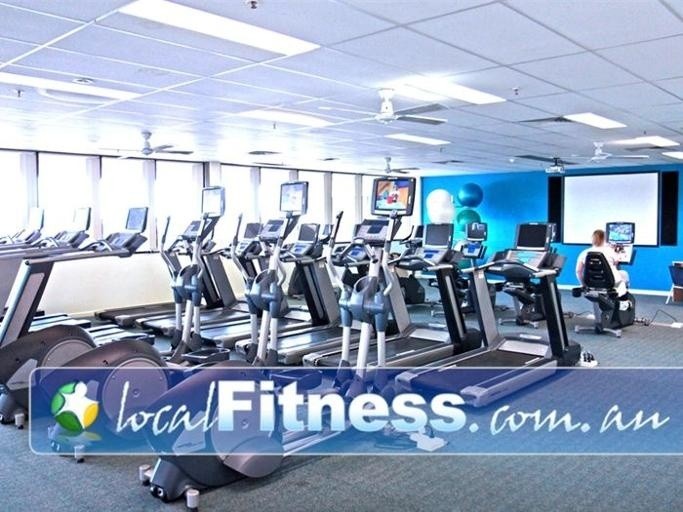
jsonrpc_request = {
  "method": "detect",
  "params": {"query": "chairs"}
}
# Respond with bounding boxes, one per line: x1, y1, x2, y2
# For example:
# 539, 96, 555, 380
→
665, 266, 683, 305
572, 252, 622, 338
498, 259, 539, 329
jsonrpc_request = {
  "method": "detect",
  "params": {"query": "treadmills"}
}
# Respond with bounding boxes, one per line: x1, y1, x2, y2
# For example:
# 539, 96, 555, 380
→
300, 222, 483, 390
394, 221, 581, 413
135, 183, 375, 369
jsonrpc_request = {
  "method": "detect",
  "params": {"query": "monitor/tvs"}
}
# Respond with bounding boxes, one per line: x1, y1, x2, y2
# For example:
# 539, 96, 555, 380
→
28, 207, 44, 229
179, 176, 636, 266
72, 207, 91, 230
125, 207, 148, 233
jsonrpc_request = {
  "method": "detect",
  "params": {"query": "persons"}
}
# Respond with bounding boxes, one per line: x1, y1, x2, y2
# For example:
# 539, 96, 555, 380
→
387, 184, 399, 204
576, 230, 630, 298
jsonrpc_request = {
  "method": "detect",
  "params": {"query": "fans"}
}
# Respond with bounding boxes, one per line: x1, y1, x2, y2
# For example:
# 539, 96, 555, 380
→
309, 88, 450, 134
559, 141, 649, 165
366, 156, 420, 175
99, 132, 193, 161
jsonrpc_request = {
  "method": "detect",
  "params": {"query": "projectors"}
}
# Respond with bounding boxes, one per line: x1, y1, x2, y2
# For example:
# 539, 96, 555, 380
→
545, 164, 565, 174
